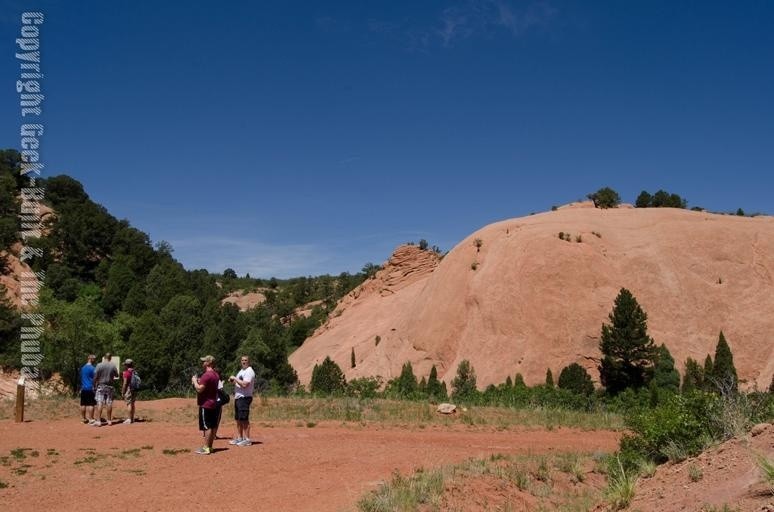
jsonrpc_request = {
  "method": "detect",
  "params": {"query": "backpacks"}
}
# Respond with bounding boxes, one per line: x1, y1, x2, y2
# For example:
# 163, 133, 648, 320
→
130, 370, 141, 390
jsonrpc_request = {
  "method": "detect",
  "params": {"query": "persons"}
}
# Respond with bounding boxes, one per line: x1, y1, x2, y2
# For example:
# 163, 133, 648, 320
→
228, 355, 256, 446
190, 354, 223, 456
92, 352, 118, 428
201, 369, 225, 440
79, 354, 96, 426
120, 358, 138, 424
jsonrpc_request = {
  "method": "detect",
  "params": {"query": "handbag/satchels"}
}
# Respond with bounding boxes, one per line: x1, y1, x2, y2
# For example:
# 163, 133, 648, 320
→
218, 387, 230, 407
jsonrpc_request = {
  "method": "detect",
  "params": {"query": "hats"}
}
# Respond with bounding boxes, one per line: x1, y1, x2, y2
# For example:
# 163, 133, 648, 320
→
122, 358, 135, 365
200, 355, 215, 362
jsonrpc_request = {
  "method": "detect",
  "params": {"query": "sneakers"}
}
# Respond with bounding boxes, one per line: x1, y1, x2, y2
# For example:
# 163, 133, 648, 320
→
229, 438, 252, 447
81, 417, 135, 427
194, 445, 213, 455
